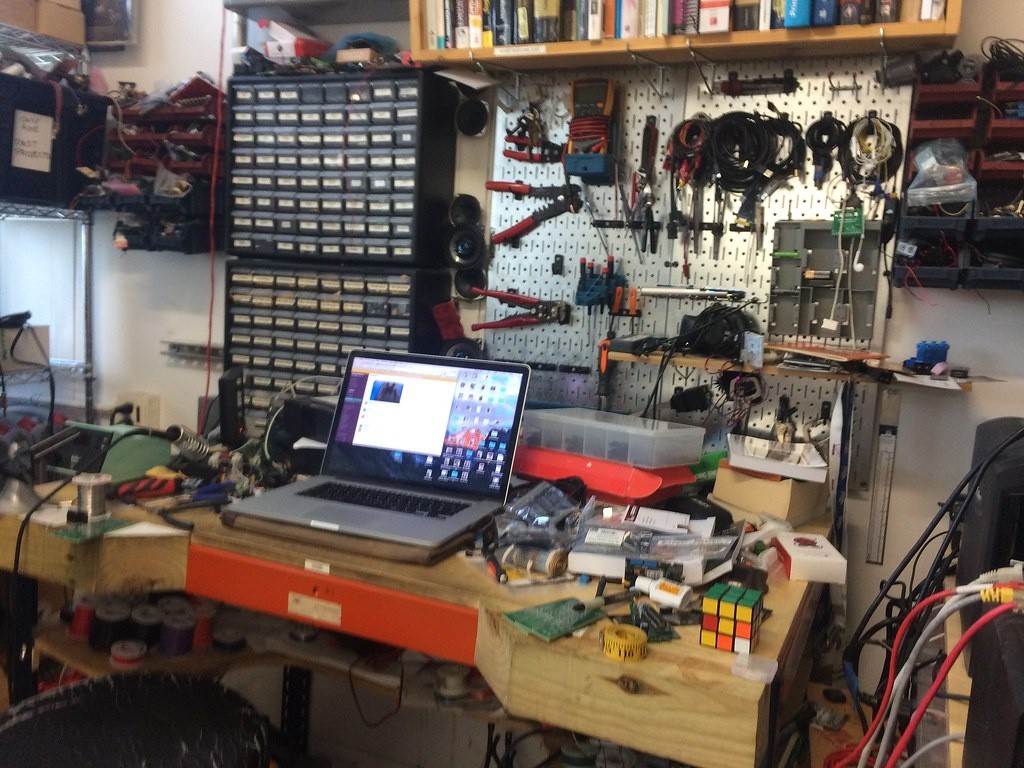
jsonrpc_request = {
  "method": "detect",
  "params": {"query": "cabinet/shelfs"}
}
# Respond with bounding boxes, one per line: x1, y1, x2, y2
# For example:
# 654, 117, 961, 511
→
0, 484, 835, 768
889, 67, 1024, 290
409, 0, 960, 73
0, 72, 93, 426
223, 67, 457, 470
104, 78, 223, 255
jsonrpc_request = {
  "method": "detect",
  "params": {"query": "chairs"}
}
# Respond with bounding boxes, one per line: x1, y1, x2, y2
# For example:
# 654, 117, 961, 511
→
0, 668, 275, 768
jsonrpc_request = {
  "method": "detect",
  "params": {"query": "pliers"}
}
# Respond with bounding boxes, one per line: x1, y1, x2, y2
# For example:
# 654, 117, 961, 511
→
502, 135, 562, 163
468, 287, 571, 332
485, 180, 584, 244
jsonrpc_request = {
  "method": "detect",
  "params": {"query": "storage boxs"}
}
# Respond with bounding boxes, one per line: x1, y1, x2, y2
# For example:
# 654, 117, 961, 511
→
712, 460, 829, 521
770, 531, 847, 587
0, 325, 49, 373
0, 0, 87, 45
512, 443, 696, 508
523, 406, 706, 470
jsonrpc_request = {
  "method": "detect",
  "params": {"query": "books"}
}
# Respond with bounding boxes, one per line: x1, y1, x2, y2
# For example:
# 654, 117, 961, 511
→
426, 0, 947, 50
568, 504, 746, 587
726, 433, 828, 483
764, 342, 890, 362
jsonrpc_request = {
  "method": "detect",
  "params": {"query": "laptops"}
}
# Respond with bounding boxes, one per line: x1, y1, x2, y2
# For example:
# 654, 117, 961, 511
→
223, 346, 531, 550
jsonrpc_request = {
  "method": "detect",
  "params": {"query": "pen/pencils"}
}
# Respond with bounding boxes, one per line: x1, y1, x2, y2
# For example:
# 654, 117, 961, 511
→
481, 546, 509, 584
570, 590, 641, 612
594, 576, 607, 597
